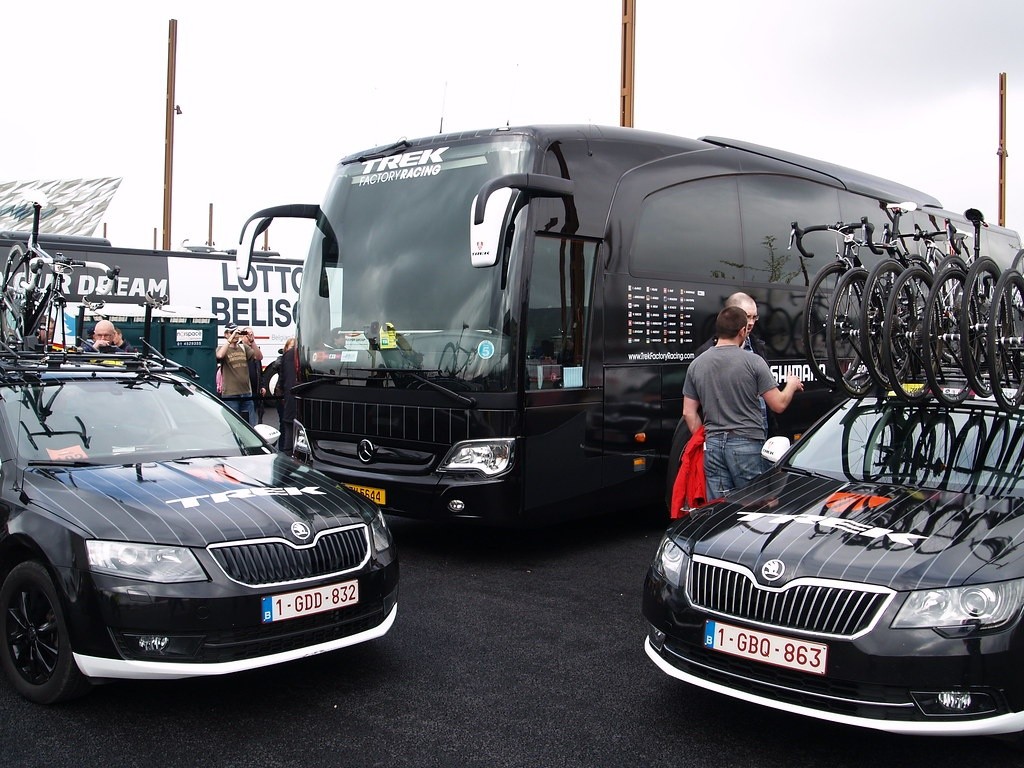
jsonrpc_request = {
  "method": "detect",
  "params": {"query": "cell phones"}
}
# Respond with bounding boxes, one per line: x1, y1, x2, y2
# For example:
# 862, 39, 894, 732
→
239, 331, 248, 335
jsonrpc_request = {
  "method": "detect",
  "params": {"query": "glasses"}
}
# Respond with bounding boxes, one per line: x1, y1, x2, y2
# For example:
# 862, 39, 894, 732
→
747, 315, 759, 321
229, 332, 233, 335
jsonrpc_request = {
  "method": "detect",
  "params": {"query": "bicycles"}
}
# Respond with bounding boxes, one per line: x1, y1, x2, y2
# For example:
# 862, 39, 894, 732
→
808, 395, 1024, 554
7, 383, 92, 451
0, 232, 121, 346
788, 201, 1024, 414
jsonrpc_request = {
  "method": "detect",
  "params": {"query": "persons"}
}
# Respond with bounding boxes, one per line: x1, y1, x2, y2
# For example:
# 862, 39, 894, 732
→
682, 306, 804, 503
37, 316, 55, 344
281, 348, 296, 457
80, 320, 124, 355
329, 328, 347, 349
694, 292, 778, 440
112, 327, 137, 353
215, 323, 263, 428
260, 338, 295, 452
238, 327, 265, 424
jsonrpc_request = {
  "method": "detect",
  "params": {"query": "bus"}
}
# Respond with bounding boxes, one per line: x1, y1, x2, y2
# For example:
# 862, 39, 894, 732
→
236, 123, 1023, 563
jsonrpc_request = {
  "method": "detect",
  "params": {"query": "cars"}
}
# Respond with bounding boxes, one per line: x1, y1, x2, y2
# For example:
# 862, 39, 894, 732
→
0, 352, 398, 705
640, 386, 1024, 739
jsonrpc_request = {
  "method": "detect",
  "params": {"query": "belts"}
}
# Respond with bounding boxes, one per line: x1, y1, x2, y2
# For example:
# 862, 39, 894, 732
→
709, 432, 744, 439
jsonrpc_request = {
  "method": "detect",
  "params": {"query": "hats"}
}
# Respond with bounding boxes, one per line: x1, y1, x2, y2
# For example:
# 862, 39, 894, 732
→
86, 325, 95, 335
224, 323, 237, 333
